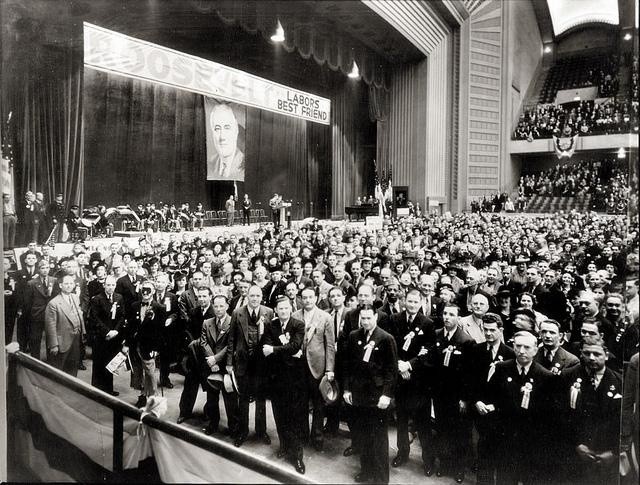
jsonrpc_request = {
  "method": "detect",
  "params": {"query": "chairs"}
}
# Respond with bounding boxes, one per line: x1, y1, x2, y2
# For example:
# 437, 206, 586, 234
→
222, 211, 228, 225
217, 211, 228, 226
234, 210, 241, 226
250, 209, 257, 223
211, 211, 221, 226
203, 216, 209, 226
240, 210, 248, 225
205, 211, 215, 226
260, 209, 268, 222
255, 209, 263, 222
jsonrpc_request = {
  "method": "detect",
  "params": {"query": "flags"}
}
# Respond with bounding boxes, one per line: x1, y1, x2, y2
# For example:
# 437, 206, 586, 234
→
0, 121, 14, 200
373, 165, 392, 212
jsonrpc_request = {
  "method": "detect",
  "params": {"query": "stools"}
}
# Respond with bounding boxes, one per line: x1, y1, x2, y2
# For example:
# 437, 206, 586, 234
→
68, 230, 78, 241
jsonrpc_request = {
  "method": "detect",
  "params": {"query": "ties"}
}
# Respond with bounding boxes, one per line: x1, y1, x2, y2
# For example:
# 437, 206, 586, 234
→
334, 309, 338, 343
545, 350, 552, 365
364, 330, 368, 346
132, 276, 136, 284
520, 367, 526, 384
529, 285, 534, 294
215, 319, 222, 341
43, 277, 47, 295
444, 332, 450, 345
251, 310, 256, 325
202, 307, 205, 315
221, 163, 227, 176
315, 286, 320, 307
158, 293, 162, 304
240, 296, 245, 307
391, 303, 399, 314
407, 315, 413, 330
487, 345, 494, 366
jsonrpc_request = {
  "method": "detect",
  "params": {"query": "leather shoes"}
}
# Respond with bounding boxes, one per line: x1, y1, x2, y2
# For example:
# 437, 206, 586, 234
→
424, 463, 433, 476
355, 472, 368, 482
234, 435, 246, 447
312, 435, 324, 451
436, 466, 448, 476
256, 432, 271, 445
161, 382, 173, 388
343, 447, 353, 456
392, 456, 409, 467
455, 471, 465, 483
78, 363, 86, 370
107, 391, 119, 396
177, 417, 186, 424
135, 396, 147, 409
295, 459, 305, 473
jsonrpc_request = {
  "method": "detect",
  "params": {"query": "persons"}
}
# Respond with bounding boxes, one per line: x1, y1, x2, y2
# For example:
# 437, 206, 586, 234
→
175, 286, 216, 425
64, 259, 86, 299
20, 239, 42, 267
429, 301, 476, 483
45, 272, 86, 378
54, 256, 70, 278
385, 289, 436, 478
291, 288, 335, 453
152, 272, 178, 388
51, 193, 68, 242
39, 244, 57, 264
67, 205, 89, 242
463, 313, 515, 485
122, 280, 166, 410
513, 308, 536, 333
3, 191, 18, 250
21, 252, 40, 279
22, 189, 34, 245
207, 105, 245, 181
225, 284, 274, 448
22, 260, 57, 359
77, 251, 89, 290
569, 316, 618, 371
4, 257, 20, 345
205, 295, 239, 438
555, 338, 623, 485
70, 243, 83, 257
622, 352, 640, 474
342, 301, 398, 485
603, 293, 628, 359
571, 290, 618, 348
511, 91, 631, 140
325, 288, 353, 439
262, 296, 306, 474
631, 56, 640, 134
587, 56, 618, 97
89, 158, 640, 320
341, 285, 388, 458
486, 331, 560, 485
33, 192, 47, 244
88, 275, 121, 397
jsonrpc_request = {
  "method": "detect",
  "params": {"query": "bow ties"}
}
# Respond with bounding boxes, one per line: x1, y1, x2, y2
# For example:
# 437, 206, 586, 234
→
141, 302, 149, 307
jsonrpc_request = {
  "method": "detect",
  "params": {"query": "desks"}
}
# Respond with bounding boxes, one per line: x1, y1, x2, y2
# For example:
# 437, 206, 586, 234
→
345, 206, 379, 221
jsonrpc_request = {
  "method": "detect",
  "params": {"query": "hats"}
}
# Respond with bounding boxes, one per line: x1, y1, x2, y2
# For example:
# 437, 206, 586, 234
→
148, 257, 158, 267
124, 352, 135, 374
515, 255, 530, 263
433, 263, 446, 271
210, 267, 226, 278
89, 252, 100, 268
446, 264, 459, 272
269, 265, 284, 272
160, 251, 168, 258
511, 309, 536, 320
133, 256, 147, 260
437, 284, 453, 292
425, 249, 436, 257
319, 376, 339, 406
301, 258, 316, 268
224, 370, 240, 395
174, 271, 187, 281
92, 259, 106, 275
496, 286, 515, 299
207, 370, 224, 390
